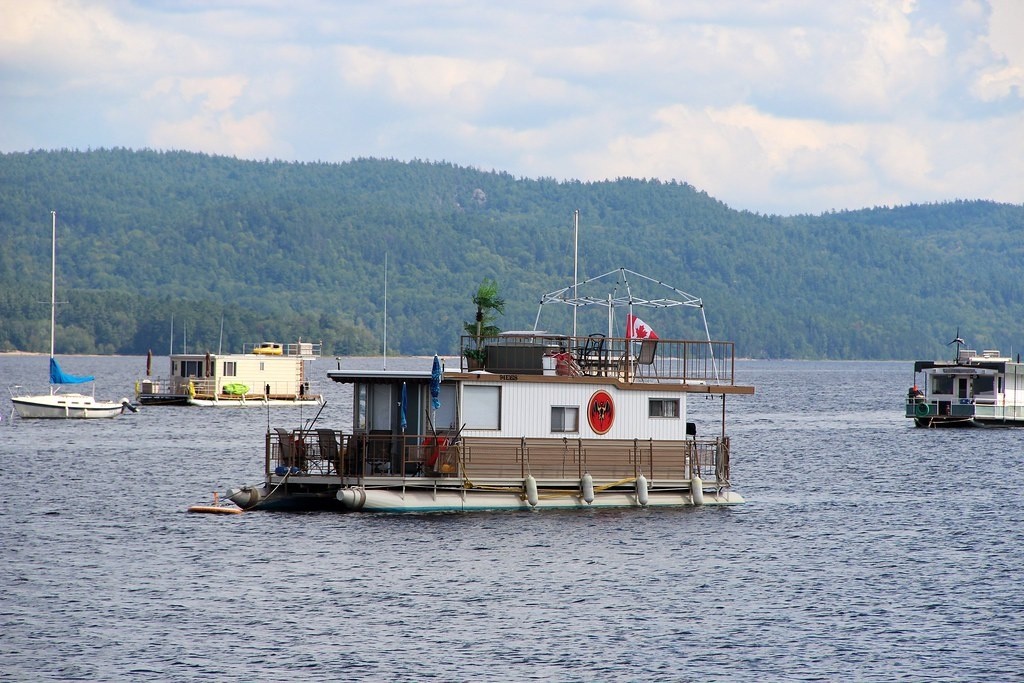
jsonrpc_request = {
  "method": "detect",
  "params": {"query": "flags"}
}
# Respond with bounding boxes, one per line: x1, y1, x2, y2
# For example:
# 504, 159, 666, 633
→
49, 356, 94, 384
626, 313, 659, 345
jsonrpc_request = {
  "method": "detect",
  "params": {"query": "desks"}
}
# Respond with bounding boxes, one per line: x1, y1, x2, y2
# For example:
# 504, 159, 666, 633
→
572, 348, 628, 376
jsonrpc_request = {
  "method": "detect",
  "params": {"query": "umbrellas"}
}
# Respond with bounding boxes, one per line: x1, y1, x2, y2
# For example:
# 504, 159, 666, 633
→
400, 381, 408, 433
146, 347, 152, 376
430, 353, 441, 436
206, 352, 211, 377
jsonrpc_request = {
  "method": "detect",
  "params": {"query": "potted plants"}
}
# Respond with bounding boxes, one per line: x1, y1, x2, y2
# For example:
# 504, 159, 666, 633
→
463, 279, 507, 372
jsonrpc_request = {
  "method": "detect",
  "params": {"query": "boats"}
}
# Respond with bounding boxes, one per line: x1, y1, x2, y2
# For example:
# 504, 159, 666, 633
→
134, 309, 327, 406
188, 490, 243, 515
904, 326, 1024, 428
224, 209, 757, 519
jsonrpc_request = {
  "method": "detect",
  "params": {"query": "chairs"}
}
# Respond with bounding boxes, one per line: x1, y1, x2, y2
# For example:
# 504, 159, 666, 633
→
617, 338, 659, 383
315, 429, 347, 476
274, 427, 323, 475
366, 429, 393, 475
573, 333, 605, 377
333, 431, 370, 476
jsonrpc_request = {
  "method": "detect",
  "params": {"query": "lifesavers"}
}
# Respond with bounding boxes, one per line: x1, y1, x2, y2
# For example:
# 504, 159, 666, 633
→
916, 402, 929, 417
421, 434, 447, 468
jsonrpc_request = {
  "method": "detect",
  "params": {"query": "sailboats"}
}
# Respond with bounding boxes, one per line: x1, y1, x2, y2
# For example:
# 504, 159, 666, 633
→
6, 211, 139, 417
